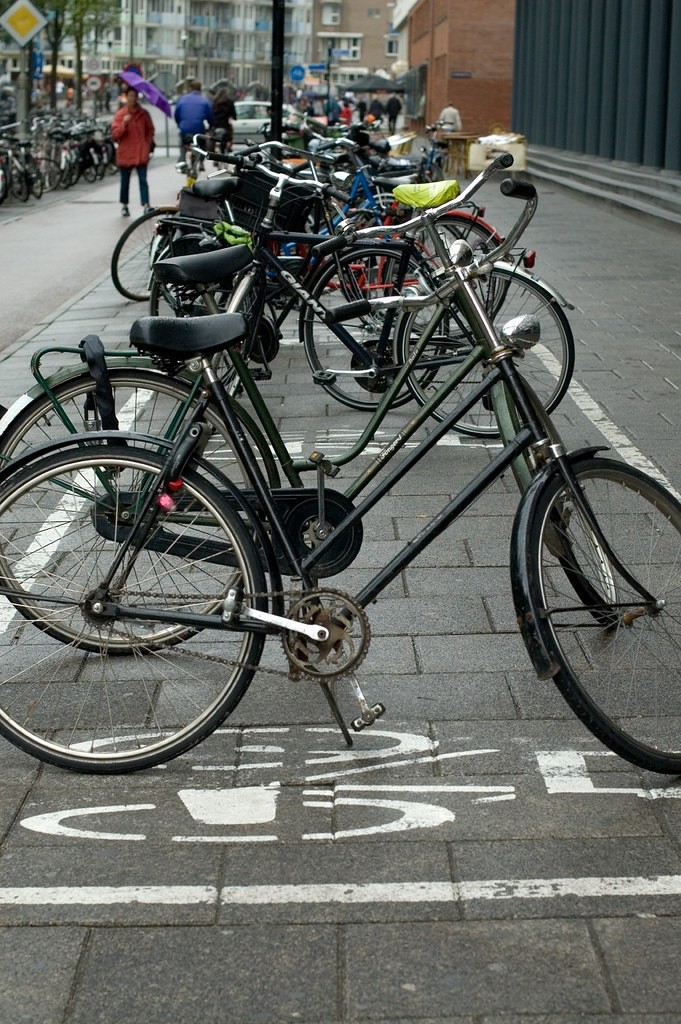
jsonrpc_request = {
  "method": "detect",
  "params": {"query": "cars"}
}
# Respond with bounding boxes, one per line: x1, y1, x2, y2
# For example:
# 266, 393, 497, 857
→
204, 102, 304, 147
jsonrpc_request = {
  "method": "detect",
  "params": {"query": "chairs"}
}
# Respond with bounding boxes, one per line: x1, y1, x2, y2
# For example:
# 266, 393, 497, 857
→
489, 122, 508, 134
386, 130, 418, 157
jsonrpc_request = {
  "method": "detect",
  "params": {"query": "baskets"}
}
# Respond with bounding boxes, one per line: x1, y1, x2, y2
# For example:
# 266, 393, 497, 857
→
232, 162, 317, 234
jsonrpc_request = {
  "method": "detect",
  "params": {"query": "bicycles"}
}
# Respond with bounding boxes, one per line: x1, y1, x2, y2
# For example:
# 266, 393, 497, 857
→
0, 105, 681, 777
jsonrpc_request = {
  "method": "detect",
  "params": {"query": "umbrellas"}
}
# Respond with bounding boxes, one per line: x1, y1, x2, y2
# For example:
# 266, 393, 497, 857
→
35, 63, 83, 80
117, 72, 171, 118
344, 74, 404, 106
292, 91, 326, 103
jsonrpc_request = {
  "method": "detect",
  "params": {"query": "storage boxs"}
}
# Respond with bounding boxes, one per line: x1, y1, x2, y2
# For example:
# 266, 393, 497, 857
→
233, 171, 315, 233
178, 192, 220, 219
468, 143, 526, 171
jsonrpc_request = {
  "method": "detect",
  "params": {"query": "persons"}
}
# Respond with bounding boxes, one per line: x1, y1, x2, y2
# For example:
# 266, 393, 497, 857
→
35, 85, 112, 113
297, 94, 402, 135
437, 101, 462, 132
174, 81, 237, 172
112, 87, 155, 216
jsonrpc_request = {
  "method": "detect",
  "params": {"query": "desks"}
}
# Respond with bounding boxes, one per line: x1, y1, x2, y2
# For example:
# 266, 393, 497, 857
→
442, 131, 479, 179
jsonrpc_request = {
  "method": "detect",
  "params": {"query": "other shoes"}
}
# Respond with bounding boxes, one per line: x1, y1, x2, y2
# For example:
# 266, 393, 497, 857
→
121, 207, 130, 216
199, 164, 205, 171
213, 161, 218, 166
143, 205, 154, 214
